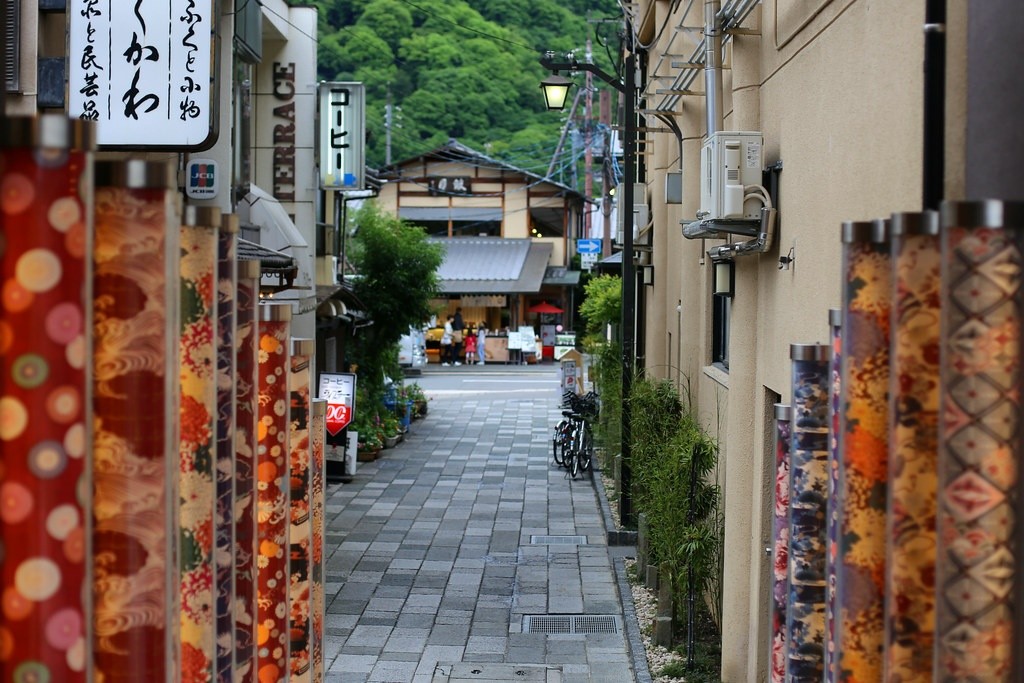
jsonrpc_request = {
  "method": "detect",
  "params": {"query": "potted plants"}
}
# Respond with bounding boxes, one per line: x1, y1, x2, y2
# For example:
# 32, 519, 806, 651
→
345, 381, 433, 462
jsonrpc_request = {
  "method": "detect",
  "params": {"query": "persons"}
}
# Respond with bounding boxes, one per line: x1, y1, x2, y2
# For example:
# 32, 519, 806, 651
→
440, 307, 487, 367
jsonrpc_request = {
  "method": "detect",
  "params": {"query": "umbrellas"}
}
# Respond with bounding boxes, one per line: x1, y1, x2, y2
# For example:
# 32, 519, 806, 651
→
527, 301, 565, 339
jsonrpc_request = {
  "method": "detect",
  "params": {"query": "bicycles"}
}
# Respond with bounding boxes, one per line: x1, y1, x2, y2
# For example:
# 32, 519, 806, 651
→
553, 389, 600, 481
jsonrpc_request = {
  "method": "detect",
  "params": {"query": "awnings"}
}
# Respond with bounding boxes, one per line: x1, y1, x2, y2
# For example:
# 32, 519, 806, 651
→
425, 237, 554, 292
543, 270, 581, 284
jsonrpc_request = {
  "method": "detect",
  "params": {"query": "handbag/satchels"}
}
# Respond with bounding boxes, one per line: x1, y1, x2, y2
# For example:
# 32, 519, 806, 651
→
441, 337, 452, 346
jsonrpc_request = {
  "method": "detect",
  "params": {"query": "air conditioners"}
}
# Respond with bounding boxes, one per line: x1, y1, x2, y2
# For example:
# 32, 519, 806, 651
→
615, 183, 648, 245
698, 130, 764, 221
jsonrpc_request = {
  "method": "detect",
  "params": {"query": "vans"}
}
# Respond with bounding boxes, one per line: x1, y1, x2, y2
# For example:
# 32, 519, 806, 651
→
344, 274, 429, 376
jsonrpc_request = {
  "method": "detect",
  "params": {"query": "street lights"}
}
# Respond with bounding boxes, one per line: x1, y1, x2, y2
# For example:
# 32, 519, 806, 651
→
539, 50, 641, 524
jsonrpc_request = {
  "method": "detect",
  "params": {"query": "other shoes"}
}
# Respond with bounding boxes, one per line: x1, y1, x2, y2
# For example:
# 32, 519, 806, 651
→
442, 362, 450, 367
477, 361, 485, 366
466, 362, 469, 365
454, 361, 462, 367
471, 361, 474, 365
